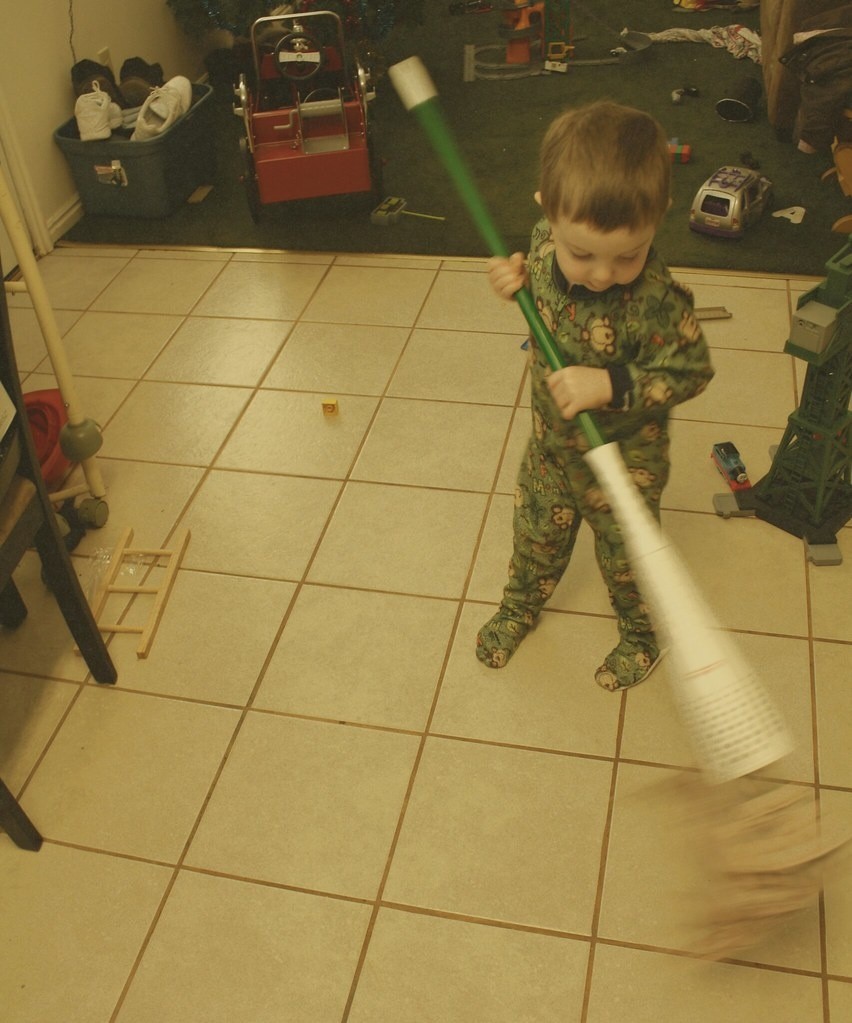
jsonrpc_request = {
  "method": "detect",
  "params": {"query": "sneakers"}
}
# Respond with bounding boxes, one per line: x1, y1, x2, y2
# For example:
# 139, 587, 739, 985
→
74, 80, 123, 142
130, 75, 192, 143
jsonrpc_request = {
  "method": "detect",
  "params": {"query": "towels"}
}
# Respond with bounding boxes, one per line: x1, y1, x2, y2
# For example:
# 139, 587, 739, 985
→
23, 388, 72, 486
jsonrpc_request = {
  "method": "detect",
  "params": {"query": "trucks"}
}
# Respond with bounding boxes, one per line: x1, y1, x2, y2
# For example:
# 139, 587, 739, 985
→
231, 11, 378, 218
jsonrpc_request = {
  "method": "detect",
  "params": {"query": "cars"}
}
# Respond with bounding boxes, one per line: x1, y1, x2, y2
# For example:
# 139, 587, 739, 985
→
688, 165, 773, 240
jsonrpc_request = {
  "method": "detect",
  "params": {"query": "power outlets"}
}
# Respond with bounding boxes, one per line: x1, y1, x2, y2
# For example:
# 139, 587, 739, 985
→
97, 47, 115, 74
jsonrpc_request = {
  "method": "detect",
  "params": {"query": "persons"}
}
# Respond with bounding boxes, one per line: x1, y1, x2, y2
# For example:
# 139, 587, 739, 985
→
474, 102, 715, 693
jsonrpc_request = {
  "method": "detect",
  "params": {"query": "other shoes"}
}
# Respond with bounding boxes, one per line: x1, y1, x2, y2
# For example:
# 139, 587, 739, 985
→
120, 57, 164, 106
70, 58, 120, 100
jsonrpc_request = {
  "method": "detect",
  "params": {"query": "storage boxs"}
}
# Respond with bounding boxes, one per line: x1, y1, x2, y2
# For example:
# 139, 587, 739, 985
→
53, 76, 215, 221
